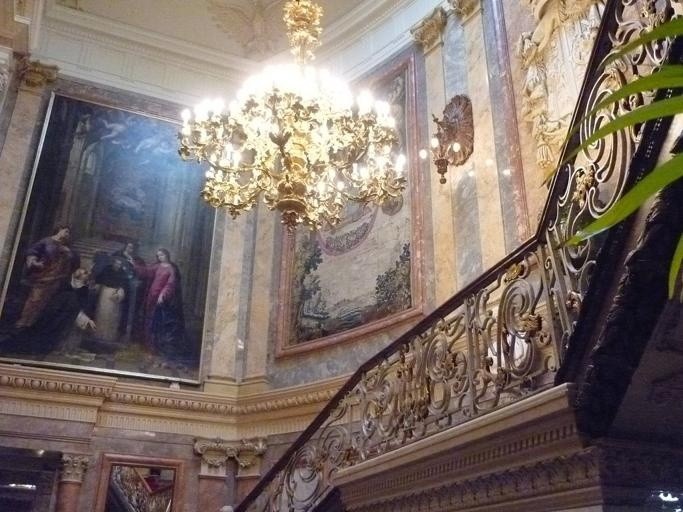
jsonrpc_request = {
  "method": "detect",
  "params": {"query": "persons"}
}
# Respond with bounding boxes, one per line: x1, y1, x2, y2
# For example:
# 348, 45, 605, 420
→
0, 219, 185, 361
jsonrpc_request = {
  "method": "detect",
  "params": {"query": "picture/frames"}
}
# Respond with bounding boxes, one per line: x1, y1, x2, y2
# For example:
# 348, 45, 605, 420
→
270, 41, 423, 361
94, 452, 188, 512
482, 0, 609, 258
0, 73, 229, 388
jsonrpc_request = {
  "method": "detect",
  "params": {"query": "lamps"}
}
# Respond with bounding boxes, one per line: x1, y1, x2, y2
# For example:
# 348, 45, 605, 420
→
176, 2, 409, 234
418, 89, 473, 187
